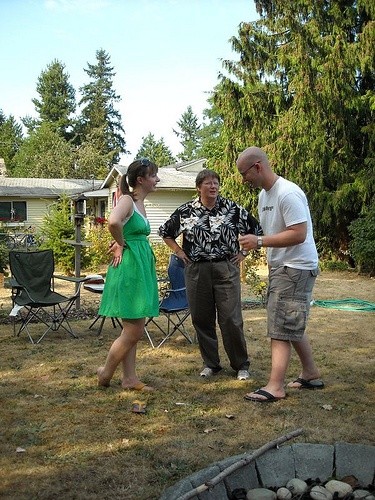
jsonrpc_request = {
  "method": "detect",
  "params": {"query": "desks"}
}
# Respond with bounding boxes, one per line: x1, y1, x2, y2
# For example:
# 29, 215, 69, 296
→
84, 283, 123, 337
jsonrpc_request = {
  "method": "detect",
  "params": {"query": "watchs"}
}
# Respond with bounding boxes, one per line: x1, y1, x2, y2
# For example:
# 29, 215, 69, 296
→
257, 235, 263, 249
240, 249, 248, 257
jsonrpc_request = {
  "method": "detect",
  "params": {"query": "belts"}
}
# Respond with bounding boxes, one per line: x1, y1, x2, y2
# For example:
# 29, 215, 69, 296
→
196, 256, 230, 263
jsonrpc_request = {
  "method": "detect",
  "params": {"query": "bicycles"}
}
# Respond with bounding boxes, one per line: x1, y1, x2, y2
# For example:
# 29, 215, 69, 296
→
0, 225, 41, 252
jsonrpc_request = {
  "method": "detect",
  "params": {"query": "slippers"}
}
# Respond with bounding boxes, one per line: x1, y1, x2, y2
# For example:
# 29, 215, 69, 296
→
244, 389, 286, 401
293, 378, 324, 388
136, 382, 148, 390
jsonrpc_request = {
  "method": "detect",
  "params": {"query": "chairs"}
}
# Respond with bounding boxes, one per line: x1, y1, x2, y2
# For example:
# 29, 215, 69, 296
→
144, 253, 193, 350
8, 248, 91, 345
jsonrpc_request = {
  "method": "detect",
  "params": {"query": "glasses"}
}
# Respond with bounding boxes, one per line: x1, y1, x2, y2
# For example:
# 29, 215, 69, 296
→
140, 159, 150, 170
241, 161, 261, 178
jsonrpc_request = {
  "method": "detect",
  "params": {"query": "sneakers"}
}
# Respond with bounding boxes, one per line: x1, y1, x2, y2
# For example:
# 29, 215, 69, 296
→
237, 370, 249, 380
200, 367, 214, 377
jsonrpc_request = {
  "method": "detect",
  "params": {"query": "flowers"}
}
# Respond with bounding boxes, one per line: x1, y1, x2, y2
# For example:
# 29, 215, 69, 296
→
0, 216, 24, 222
94, 216, 105, 223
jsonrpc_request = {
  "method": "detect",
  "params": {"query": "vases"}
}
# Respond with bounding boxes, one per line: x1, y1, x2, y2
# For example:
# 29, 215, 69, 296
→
0, 223, 24, 227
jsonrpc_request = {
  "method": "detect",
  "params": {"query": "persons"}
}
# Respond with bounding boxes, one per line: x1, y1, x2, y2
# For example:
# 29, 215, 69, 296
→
236, 146, 326, 403
97, 159, 160, 394
156, 170, 264, 381
